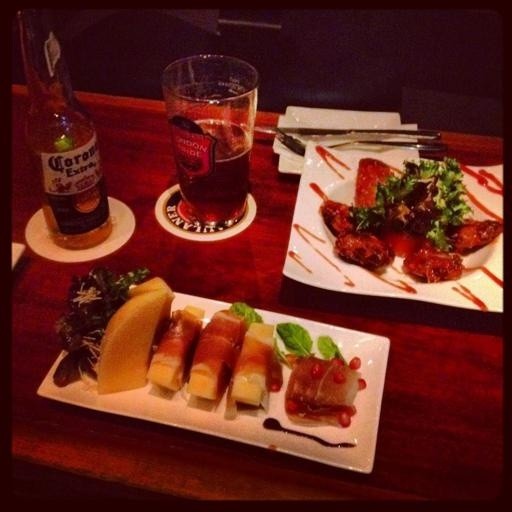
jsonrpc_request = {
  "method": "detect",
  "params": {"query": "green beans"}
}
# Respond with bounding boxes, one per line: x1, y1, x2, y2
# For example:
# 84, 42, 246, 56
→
285, 351, 367, 427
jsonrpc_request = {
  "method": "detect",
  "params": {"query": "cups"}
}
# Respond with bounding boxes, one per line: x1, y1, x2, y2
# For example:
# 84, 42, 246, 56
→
160, 54, 261, 223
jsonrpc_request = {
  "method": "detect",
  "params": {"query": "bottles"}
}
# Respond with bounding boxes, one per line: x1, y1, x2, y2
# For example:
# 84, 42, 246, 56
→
15, 10, 115, 254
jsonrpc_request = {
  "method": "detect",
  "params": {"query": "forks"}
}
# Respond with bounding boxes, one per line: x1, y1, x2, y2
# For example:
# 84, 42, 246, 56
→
274, 128, 448, 162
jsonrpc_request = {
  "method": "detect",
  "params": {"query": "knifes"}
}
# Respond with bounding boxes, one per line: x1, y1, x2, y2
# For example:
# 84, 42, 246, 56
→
274, 124, 439, 137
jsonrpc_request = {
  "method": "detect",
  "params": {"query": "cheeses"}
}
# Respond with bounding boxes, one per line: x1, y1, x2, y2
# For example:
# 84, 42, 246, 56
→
97, 277, 274, 409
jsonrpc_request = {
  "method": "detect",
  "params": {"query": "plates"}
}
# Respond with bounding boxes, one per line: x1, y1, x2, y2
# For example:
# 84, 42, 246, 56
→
36, 283, 390, 478
280, 138, 504, 319
277, 106, 403, 177
